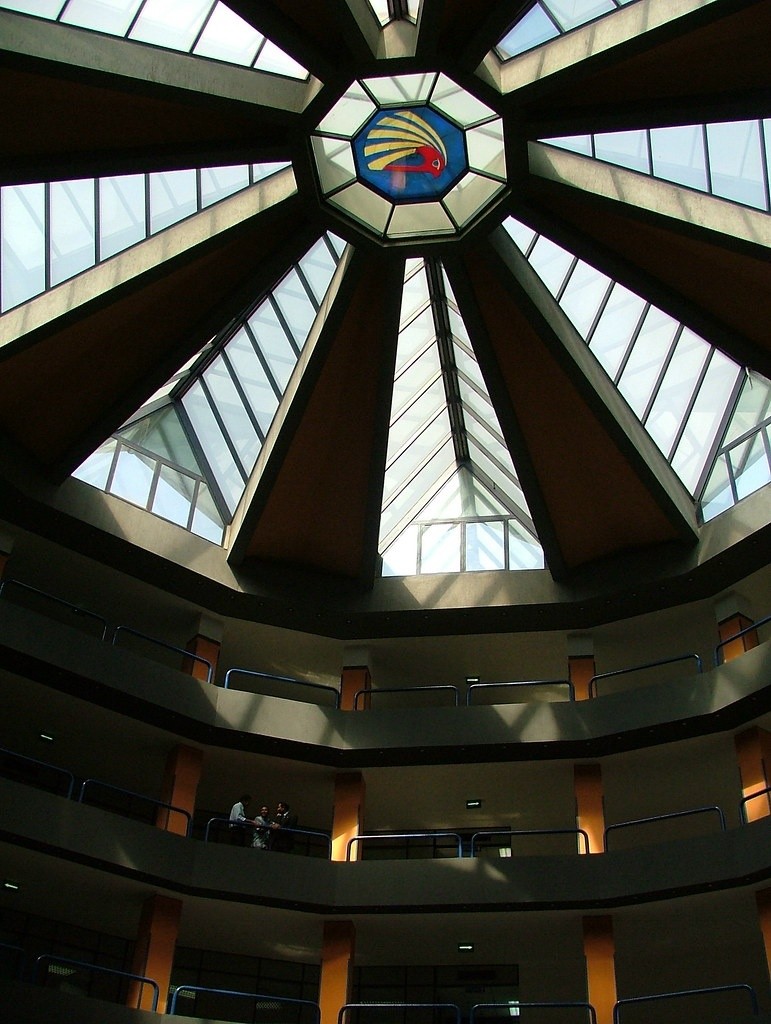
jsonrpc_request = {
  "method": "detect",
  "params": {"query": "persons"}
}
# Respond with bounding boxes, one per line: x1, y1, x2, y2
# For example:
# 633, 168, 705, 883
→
227, 794, 253, 849
268, 799, 296, 854
250, 805, 274, 846
